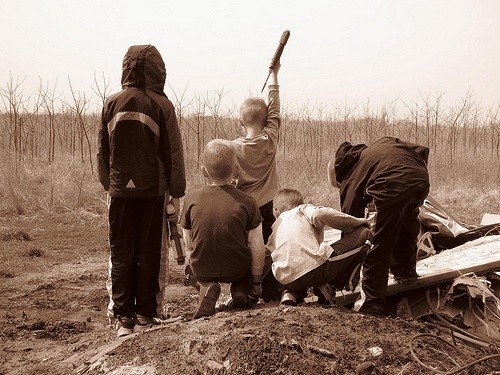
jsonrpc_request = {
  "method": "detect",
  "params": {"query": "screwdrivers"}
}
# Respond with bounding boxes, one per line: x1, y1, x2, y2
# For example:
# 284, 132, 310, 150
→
261, 30, 290, 94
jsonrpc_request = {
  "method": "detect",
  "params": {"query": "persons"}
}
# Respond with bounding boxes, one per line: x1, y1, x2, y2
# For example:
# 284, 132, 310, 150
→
327, 135, 430, 320
180, 138, 265, 320
260, 189, 374, 306
230, 58, 282, 306
95, 45, 187, 337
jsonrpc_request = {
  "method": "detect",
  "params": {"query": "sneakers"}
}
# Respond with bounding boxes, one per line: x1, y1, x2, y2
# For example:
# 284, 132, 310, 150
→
313, 283, 336, 305
135, 315, 163, 332
115, 316, 132, 336
230, 285, 248, 307
194, 282, 221, 317
281, 290, 296, 306
